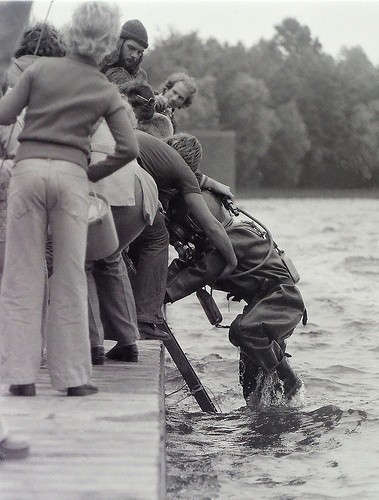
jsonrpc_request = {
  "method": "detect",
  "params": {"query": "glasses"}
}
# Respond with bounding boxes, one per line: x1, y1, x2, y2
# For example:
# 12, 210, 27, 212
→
137, 94, 158, 108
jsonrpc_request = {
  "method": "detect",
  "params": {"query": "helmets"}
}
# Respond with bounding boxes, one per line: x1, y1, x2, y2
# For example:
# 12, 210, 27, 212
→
168, 188, 234, 251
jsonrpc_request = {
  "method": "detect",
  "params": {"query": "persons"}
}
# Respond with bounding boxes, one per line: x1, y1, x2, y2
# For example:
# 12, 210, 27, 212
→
0, 1, 139, 396
84, 19, 237, 364
0, 22, 66, 362
165, 192, 307, 403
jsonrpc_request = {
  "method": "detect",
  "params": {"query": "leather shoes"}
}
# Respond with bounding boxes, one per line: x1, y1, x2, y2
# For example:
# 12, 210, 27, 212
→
137, 322, 172, 340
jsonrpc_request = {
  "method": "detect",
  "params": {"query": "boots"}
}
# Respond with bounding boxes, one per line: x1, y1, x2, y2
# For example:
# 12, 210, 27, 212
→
273, 355, 302, 402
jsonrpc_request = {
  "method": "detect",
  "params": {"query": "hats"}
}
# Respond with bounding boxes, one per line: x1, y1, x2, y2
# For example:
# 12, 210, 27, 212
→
121, 19, 150, 49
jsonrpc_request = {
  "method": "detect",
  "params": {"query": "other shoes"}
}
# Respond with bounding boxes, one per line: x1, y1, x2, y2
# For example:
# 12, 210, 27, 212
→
106, 345, 139, 362
9, 382, 37, 397
68, 381, 98, 396
91, 346, 106, 366
